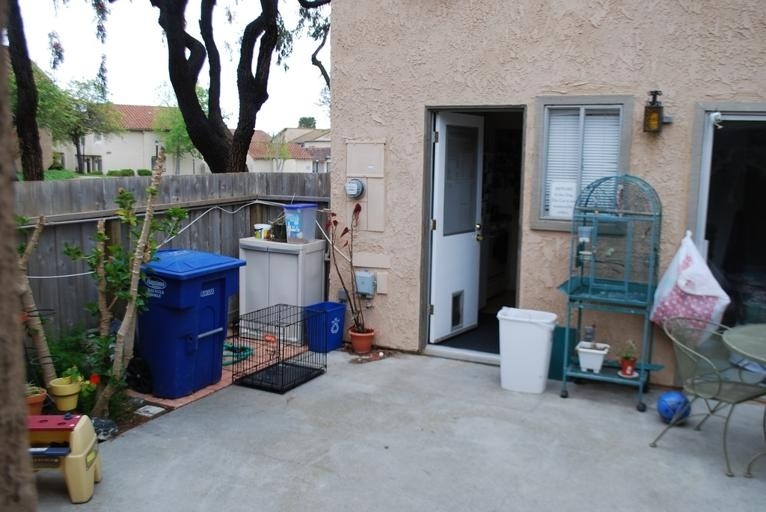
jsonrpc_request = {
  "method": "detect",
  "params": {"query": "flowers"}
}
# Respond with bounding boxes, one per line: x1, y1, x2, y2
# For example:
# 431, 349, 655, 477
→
323, 202, 366, 331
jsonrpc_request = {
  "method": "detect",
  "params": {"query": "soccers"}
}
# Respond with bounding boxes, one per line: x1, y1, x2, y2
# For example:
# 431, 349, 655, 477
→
657, 391, 690, 425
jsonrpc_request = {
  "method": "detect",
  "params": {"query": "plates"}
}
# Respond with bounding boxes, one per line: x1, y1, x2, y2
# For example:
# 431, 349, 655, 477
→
617, 370, 640, 379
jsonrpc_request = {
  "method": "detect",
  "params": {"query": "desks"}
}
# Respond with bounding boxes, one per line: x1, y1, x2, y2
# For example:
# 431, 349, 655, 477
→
723, 322, 766, 478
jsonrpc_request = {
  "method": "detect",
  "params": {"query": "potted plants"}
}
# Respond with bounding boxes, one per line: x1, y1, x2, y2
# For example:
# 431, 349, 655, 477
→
619, 338, 640, 377
48, 364, 85, 412
25, 376, 47, 415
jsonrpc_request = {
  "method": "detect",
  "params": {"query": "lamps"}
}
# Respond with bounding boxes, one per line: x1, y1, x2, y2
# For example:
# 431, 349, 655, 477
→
642, 88, 673, 135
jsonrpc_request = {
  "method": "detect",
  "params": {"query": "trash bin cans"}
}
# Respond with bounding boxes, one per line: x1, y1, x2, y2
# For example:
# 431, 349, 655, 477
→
113, 248, 247, 400
496, 306, 558, 394
304, 302, 347, 353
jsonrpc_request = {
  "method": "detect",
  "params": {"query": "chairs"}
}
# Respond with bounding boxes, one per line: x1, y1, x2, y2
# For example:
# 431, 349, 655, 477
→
650, 315, 766, 479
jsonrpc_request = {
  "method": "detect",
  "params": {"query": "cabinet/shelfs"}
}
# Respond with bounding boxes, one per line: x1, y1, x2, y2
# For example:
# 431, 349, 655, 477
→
556, 173, 664, 412
237, 235, 327, 348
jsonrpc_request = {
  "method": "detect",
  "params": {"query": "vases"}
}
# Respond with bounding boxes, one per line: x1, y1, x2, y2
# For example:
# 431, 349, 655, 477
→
347, 327, 375, 355
574, 340, 611, 374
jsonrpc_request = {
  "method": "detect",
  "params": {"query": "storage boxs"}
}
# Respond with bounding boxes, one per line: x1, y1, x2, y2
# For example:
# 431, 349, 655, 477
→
284, 203, 318, 245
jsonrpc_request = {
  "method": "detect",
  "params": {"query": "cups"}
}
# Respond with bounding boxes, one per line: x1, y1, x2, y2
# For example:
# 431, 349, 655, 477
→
579, 252, 591, 262
579, 226, 592, 243
